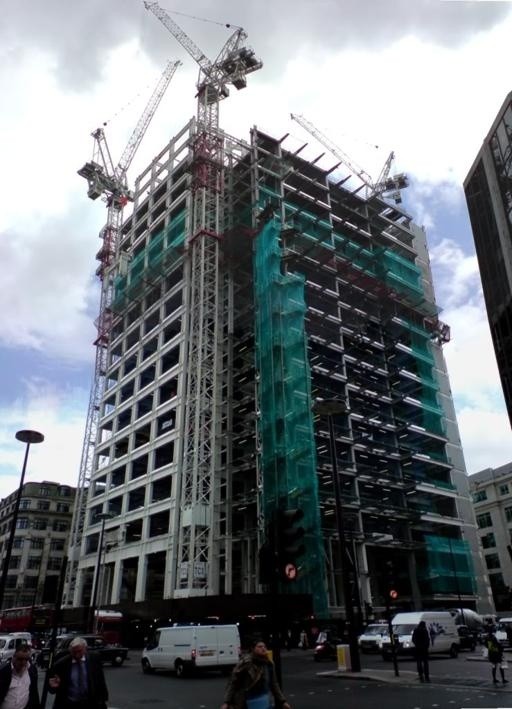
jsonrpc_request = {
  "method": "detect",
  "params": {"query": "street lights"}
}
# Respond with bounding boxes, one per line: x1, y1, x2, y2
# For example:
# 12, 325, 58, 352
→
93, 509, 115, 607
1, 428, 48, 601
310, 395, 362, 671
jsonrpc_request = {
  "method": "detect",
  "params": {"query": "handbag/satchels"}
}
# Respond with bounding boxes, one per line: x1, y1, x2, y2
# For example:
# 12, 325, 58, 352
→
481, 647, 488, 658
246, 693, 275, 709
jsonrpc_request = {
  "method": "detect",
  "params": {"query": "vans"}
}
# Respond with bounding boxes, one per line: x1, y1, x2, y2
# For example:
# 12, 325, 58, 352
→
10, 632, 32, 646
493, 617, 511, 648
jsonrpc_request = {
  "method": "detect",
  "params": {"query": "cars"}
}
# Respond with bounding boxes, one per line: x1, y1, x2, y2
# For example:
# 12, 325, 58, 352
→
0, 631, 38, 669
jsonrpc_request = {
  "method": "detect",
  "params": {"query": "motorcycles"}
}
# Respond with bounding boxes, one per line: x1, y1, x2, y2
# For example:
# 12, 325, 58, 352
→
310, 630, 346, 663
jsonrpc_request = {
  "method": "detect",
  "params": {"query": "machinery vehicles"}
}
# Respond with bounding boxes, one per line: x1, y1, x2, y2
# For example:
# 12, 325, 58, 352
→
452, 607, 484, 652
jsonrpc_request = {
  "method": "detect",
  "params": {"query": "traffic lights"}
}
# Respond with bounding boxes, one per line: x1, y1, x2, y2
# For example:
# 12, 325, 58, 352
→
377, 558, 399, 600
274, 506, 306, 583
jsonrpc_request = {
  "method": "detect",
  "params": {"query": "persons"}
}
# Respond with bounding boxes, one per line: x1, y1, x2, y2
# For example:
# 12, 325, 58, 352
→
0, 646, 41, 709
287, 628, 293, 651
47, 636, 109, 709
299, 629, 309, 650
412, 620, 432, 683
484, 627, 508, 684
220, 638, 290, 708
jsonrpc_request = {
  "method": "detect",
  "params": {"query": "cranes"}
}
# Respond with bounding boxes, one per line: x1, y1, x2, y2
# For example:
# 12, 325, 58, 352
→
144, 1, 263, 587
63, 59, 183, 608
289, 111, 414, 204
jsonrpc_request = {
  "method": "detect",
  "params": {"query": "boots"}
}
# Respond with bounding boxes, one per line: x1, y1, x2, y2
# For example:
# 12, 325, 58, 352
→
492, 666, 498, 682
500, 666, 509, 683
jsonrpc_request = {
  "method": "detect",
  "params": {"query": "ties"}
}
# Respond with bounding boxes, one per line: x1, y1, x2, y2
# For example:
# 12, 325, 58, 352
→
76, 659, 85, 698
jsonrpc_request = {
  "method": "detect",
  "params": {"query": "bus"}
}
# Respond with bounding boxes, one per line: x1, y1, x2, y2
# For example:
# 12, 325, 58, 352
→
1, 603, 57, 637
56, 609, 129, 643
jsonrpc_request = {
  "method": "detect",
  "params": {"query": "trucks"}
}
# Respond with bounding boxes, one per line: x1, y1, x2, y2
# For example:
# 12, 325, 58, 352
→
129, 617, 202, 646
139, 622, 245, 679
381, 610, 461, 663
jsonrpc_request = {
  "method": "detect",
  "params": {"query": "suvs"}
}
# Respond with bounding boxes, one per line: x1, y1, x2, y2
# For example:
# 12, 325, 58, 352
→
36, 634, 133, 672
358, 619, 388, 654
